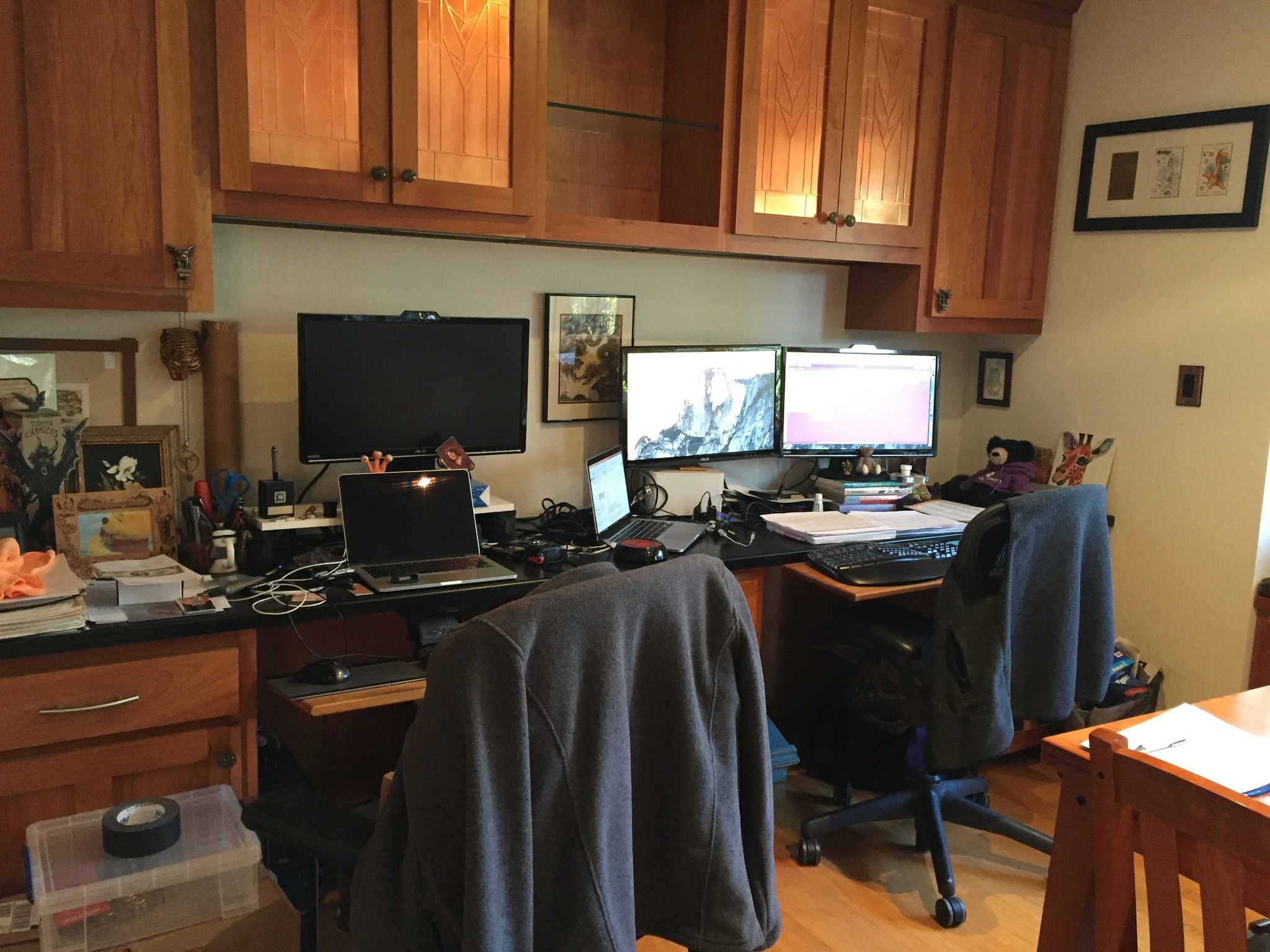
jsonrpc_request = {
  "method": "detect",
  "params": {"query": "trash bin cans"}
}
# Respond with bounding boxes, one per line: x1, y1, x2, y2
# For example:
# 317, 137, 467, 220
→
1061, 696, 1149, 730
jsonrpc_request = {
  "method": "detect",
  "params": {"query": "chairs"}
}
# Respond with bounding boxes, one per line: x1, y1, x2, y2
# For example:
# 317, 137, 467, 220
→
1089, 728, 1269, 952
797, 484, 1115, 927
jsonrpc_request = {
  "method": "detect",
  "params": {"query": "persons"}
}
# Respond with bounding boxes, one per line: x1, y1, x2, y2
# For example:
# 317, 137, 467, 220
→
445, 448, 472, 469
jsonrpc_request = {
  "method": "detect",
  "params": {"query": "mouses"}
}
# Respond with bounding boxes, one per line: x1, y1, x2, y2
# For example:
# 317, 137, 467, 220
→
289, 659, 351, 684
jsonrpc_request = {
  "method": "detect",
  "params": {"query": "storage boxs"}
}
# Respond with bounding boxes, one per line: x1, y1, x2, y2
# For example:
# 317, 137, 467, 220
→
1107, 647, 1135, 692
0, 875, 302, 952
25, 783, 263, 952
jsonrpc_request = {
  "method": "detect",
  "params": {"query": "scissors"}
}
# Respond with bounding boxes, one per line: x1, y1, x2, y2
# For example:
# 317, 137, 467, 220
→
211, 469, 250, 515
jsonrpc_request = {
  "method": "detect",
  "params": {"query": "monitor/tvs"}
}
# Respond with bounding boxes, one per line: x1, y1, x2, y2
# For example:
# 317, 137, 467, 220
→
295, 311, 528, 473
619, 342, 782, 471
777, 347, 941, 480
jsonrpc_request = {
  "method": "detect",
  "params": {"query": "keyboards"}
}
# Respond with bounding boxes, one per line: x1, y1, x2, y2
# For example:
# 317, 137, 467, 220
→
805, 540, 959, 587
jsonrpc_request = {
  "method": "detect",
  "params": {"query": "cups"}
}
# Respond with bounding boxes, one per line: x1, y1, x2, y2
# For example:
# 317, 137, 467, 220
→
208, 529, 239, 574
322, 501, 339, 518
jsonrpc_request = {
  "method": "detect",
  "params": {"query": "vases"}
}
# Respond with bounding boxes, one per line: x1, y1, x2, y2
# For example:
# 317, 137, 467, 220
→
64, 425, 188, 558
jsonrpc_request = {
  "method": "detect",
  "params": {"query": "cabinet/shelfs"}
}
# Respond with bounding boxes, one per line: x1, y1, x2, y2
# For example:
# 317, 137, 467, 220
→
845, 0, 1085, 334
661, 1, 949, 265
1, 1, 213, 313
207, 1, 548, 241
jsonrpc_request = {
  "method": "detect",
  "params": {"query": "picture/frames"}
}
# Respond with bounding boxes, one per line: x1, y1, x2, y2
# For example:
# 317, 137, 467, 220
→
977, 351, 1014, 408
52, 487, 179, 580
1073, 104, 1270, 231
0, 338, 139, 428
544, 293, 636, 423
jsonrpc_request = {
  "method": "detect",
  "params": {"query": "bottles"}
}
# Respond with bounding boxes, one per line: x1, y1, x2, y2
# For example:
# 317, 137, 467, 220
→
813, 493, 823, 512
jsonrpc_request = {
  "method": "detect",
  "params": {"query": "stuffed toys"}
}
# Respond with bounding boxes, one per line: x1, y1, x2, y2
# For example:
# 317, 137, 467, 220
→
939, 435, 1035, 511
856, 446, 881, 476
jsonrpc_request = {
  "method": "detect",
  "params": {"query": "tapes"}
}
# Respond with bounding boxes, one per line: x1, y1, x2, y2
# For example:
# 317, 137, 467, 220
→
102, 797, 181, 858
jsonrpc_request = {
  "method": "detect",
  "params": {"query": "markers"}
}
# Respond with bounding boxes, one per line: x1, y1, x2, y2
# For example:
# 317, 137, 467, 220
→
195, 480, 212, 520
182, 501, 195, 542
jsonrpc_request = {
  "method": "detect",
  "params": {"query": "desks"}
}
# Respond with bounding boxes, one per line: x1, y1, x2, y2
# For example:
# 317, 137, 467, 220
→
1037, 684, 1269, 951
1, 487, 1115, 655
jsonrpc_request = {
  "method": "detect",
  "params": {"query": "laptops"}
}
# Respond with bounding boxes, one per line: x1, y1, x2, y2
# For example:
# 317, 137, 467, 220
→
338, 469, 518, 594
586, 442, 709, 551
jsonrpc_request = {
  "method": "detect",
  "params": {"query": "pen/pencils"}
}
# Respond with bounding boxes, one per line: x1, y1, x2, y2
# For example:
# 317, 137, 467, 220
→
199, 499, 211, 520
271, 446, 278, 481
772, 529, 804, 541
184, 497, 214, 544
232, 498, 248, 550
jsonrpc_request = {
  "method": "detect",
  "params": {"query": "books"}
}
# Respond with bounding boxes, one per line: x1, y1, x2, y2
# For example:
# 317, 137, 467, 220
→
0, 553, 87, 641
760, 473, 985, 545
